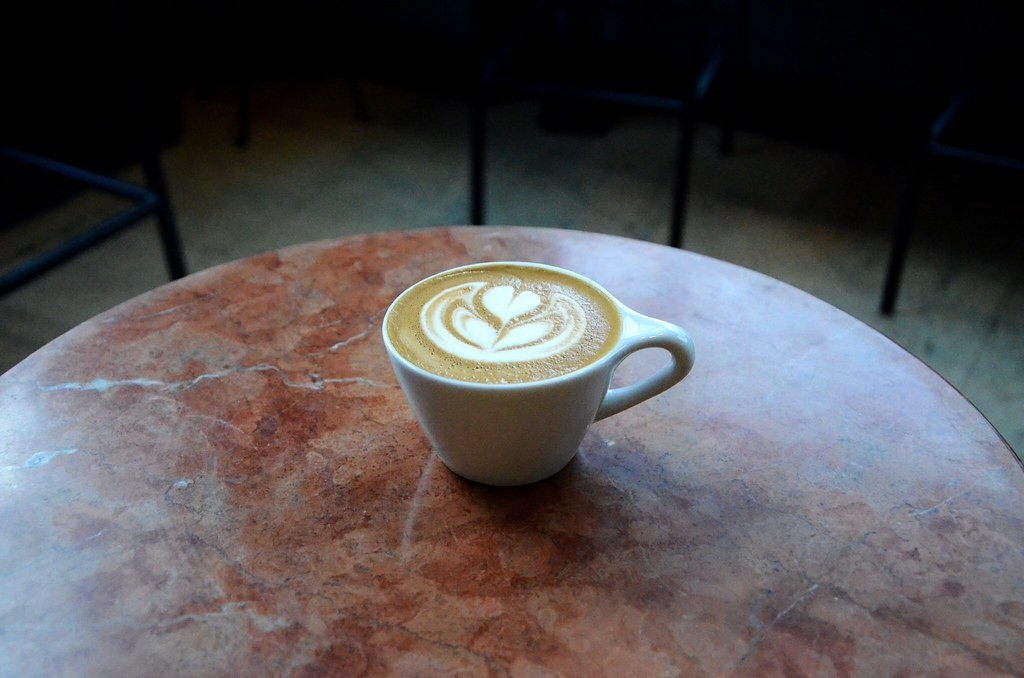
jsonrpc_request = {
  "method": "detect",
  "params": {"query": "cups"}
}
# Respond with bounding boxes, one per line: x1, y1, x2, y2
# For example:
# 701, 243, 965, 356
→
382, 261, 696, 487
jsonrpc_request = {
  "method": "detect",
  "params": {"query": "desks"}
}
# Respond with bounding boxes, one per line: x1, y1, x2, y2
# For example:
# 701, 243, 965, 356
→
0, 222, 1024, 678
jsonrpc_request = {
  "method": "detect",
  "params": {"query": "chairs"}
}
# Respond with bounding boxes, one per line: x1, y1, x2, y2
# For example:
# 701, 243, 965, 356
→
0, 0, 1024, 314
